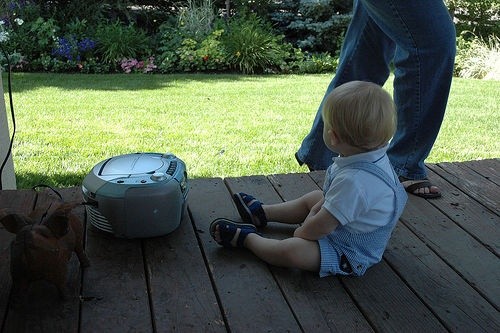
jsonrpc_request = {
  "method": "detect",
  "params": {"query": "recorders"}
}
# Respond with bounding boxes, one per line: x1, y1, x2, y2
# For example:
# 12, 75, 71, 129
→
82, 151, 190, 240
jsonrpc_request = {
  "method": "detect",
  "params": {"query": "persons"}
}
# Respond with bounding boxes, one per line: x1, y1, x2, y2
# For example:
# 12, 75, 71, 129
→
295, 0, 456, 198
209, 80, 409, 279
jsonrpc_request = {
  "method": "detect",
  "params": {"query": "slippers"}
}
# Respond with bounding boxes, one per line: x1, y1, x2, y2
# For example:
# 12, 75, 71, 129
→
398, 173, 444, 200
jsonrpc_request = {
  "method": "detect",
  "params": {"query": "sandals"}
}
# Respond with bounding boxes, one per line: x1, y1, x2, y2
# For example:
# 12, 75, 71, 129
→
209, 218, 258, 249
234, 190, 269, 228
